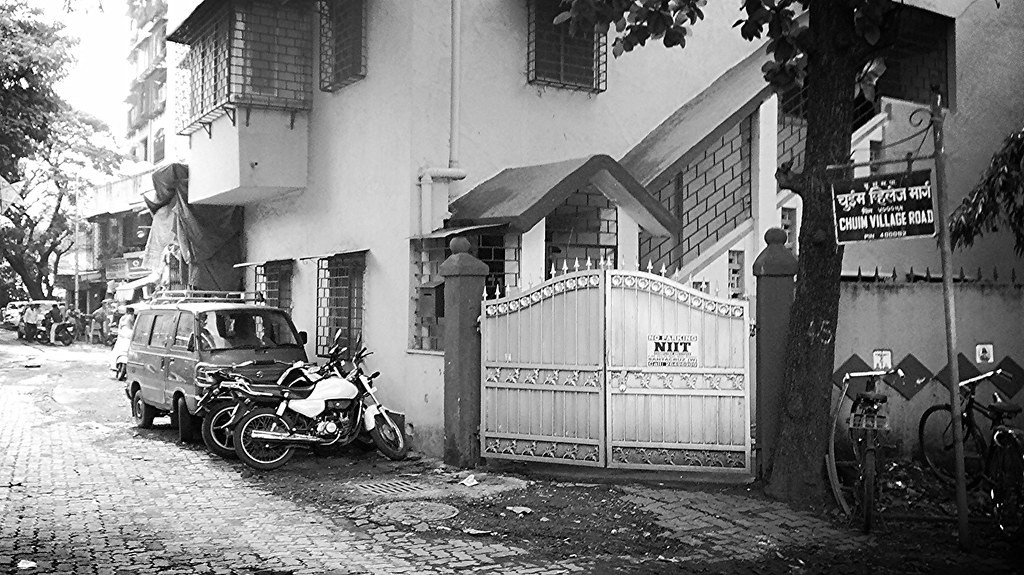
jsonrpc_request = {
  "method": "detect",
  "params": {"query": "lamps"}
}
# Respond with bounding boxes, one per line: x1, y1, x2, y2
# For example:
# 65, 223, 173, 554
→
137, 226, 152, 238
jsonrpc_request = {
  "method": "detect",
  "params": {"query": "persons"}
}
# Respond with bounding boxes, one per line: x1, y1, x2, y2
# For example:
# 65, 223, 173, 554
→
19, 298, 138, 344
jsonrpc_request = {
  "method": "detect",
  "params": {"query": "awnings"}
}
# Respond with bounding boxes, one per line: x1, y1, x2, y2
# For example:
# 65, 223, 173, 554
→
114, 278, 156, 301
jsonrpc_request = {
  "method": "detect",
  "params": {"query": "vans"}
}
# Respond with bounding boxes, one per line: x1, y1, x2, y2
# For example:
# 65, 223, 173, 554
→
124, 289, 311, 445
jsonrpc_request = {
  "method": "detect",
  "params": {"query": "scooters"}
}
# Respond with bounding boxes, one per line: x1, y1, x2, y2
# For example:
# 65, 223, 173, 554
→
35, 309, 120, 347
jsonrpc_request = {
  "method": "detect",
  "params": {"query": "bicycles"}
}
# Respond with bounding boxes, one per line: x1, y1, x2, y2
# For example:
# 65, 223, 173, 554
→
919, 369, 1024, 544
842, 367, 905, 531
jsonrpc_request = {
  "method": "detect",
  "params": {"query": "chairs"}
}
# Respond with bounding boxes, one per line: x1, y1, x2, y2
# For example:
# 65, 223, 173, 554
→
232, 318, 260, 347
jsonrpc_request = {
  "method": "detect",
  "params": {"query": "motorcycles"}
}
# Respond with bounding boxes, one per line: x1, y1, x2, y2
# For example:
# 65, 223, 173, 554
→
196, 325, 409, 471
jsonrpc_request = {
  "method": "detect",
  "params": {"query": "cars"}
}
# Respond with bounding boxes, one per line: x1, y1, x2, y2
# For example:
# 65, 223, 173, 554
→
17, 300, 62, 341
1, 301, 35, 330
